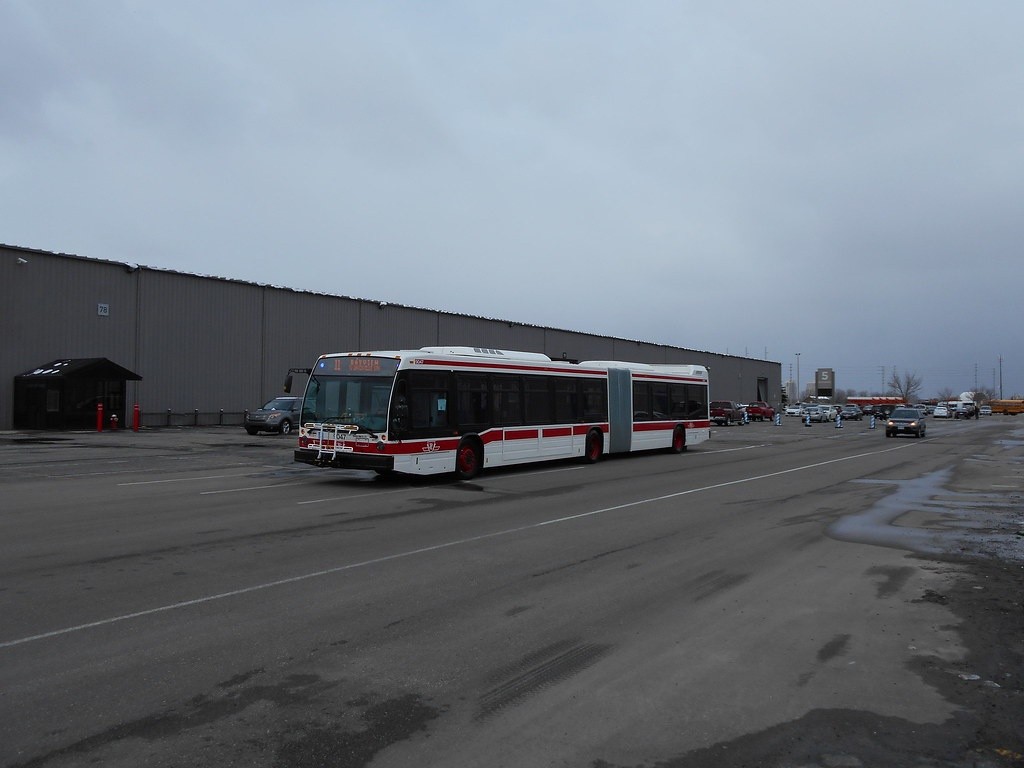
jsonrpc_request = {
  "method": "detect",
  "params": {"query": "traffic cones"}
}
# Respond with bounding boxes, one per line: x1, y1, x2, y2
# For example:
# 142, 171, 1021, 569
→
743, 412, 750, 424
866, 415, 878, 430
773, 413, 782, 426
835, 414, 844, 428
805, 413, 812, 427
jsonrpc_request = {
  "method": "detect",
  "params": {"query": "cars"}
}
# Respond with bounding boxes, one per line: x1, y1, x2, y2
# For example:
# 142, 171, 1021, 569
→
817, 404, 838, 422
886, 408, 926, 437
244, 396, 314, 434
784, 398, 992, 418
802, 407, 827, 423
839, 408, 864, 421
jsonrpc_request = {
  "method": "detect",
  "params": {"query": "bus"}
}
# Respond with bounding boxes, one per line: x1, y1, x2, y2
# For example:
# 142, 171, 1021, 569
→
283, 343, 713, 481
988, 399, 1024, 415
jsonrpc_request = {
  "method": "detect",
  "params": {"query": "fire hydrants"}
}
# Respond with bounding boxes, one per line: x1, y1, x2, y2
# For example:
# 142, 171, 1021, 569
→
110, 414, 119, 431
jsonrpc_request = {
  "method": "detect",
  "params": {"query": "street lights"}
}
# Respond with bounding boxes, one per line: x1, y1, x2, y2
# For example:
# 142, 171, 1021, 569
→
794, 352, 801, 402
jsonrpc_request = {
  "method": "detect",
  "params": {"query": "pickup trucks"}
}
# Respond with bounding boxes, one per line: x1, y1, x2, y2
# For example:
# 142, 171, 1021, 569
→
708, 400, 744, 426
738, 401, 775, 421
